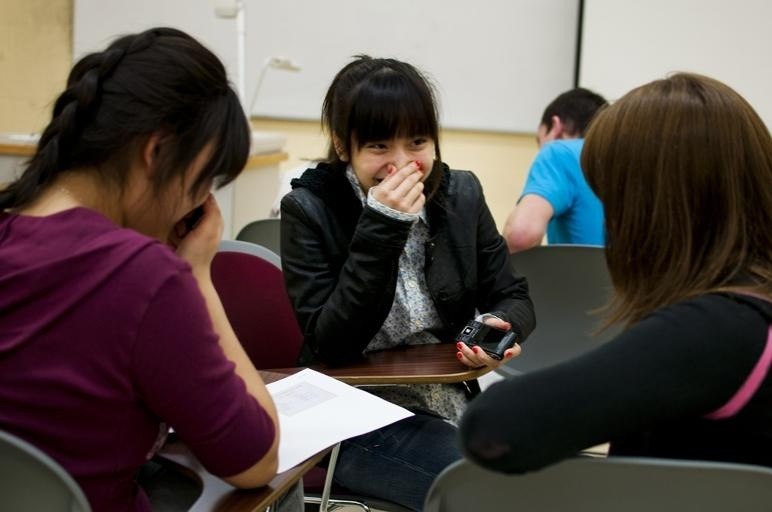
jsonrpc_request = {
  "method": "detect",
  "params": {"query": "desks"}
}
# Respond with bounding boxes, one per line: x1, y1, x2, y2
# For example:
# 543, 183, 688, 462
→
256, 340, 494, 510
0, 140, 288, 242
142, 369, 342, 511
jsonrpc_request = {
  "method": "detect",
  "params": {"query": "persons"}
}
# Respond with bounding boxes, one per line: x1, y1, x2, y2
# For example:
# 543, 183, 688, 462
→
0, 28, 279, 512
460, 70, 771, 476
280, 55, 537, 511
503, 87, 611, 252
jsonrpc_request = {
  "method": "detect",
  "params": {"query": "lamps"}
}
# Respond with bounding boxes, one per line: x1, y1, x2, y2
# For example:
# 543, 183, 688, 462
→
214, 1, 299, 120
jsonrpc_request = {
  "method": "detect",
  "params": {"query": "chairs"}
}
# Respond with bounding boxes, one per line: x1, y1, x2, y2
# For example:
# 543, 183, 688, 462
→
235, 219, 283, 262
0, 431, 94, 511
423, 451, 772, 512
209, 240, 400, 509
490, 242, 633, 378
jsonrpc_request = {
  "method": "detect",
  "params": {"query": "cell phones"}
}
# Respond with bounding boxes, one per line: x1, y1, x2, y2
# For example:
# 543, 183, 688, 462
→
456, 320, 519, 361
